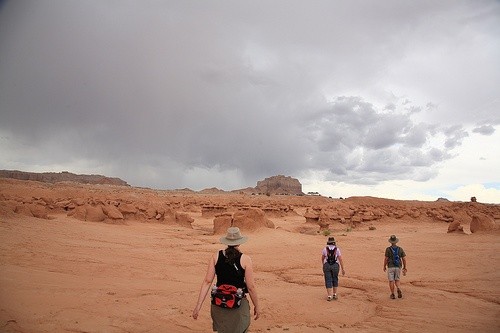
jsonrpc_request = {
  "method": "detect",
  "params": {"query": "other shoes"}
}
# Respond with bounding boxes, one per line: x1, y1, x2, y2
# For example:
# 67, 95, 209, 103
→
397, 288, 402, 298
391, 294, 395, 298
327, 296, 332, 300
333, 294, 338, 299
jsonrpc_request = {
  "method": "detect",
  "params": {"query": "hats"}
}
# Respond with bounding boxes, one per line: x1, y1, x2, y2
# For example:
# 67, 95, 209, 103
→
327, 237, 336, 243
389, 235, 399, 244
220, 228, 248, 246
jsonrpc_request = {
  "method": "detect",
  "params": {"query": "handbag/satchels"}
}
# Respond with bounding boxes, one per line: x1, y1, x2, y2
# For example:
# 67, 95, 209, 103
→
211, 285, 247, 309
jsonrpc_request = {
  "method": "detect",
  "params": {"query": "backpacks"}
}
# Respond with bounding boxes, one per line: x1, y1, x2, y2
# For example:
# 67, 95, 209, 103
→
326, 247, 337, 265
390, 246, 400, 266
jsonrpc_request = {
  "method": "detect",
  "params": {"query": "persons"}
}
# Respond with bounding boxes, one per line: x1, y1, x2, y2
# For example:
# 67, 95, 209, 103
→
383, 235, 407, 299
193, 227, 260, 333
321, 237, 345, 301
